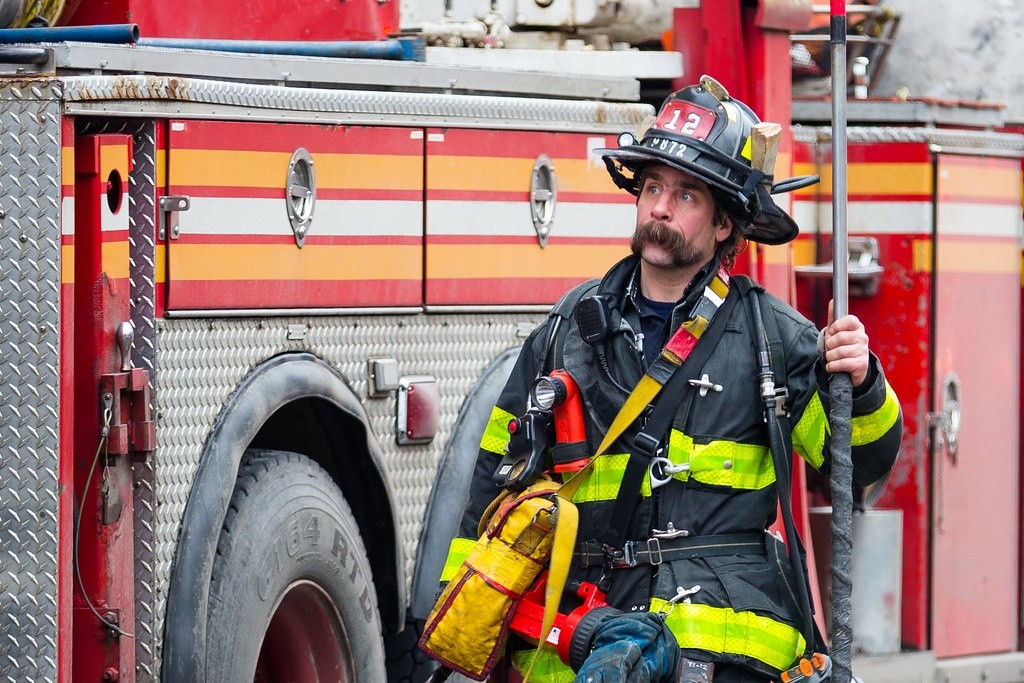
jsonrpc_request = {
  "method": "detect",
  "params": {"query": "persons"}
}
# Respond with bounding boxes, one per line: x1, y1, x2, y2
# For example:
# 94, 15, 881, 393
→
417, 73, 903, 683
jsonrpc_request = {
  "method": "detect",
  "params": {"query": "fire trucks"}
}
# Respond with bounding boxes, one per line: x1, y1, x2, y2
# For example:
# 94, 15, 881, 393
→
1, 1, 1024, 682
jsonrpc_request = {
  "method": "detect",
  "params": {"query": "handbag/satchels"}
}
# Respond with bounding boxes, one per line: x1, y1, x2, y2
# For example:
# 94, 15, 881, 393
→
417, 460, 580, 682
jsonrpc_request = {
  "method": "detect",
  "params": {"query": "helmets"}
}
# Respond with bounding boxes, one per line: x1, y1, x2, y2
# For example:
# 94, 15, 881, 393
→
591, 75, 821, 246
